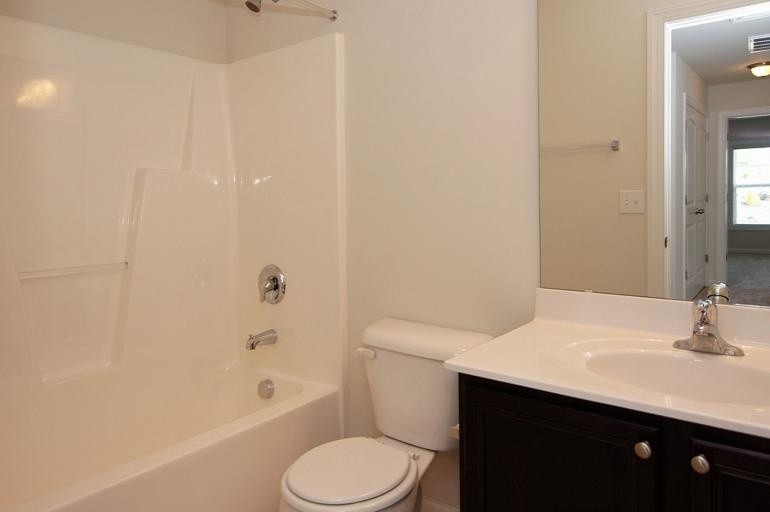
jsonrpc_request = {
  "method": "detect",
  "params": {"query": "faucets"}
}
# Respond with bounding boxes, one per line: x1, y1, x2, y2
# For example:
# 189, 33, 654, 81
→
692, 299, 719, 354
694, 282, 730, 306
246, 329, 279, 350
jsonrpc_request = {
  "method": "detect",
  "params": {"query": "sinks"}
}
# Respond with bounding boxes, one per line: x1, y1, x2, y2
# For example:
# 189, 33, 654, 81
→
564, 339, 769, 409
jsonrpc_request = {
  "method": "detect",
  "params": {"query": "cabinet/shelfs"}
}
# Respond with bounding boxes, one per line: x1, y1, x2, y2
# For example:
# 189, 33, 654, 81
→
459, 372, 770, 512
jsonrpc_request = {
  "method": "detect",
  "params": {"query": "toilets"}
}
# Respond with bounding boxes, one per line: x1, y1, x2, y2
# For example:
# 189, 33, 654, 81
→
277, 316, 493, 512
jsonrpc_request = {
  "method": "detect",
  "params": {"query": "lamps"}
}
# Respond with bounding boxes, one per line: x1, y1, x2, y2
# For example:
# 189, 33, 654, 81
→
747, 61, 770, 81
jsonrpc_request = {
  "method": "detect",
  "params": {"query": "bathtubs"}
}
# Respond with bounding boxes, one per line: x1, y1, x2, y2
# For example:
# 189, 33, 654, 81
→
1, 361, 337, 511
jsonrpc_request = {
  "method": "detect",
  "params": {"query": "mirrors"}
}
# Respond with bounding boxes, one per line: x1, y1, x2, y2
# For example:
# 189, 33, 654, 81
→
647, 0, 770, 306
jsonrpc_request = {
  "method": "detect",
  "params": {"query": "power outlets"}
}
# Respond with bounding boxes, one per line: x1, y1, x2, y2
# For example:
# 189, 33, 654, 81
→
619, 190, 643, 214
